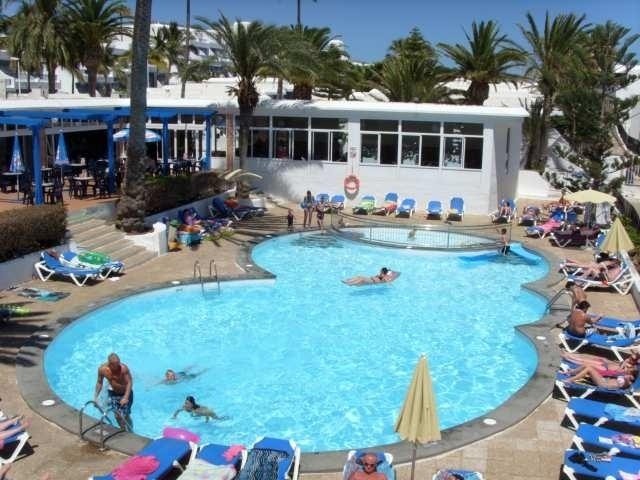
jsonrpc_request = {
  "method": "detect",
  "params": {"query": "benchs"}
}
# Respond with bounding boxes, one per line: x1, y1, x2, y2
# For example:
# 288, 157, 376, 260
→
174, 442, 248, 480
90, 438, 198, 480
341, 450, 393, 479
431, 468, 483, 480
237, 438, 301, 480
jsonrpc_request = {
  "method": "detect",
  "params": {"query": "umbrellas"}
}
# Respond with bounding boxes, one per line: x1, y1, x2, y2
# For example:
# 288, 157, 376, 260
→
396, 352, 441, 480
113, 125, 162, 143
56, 128, 70, 184
9, 130, 25, 200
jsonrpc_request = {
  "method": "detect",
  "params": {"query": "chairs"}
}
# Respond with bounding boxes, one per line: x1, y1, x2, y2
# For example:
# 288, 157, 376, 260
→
489, 199, 637, 295
177, 203, 267, 231
34, 242, 125, 286
302, 192, 465, 222
5, 156, 194, 203
554, 313, 639, 479
1, 416, 30, 478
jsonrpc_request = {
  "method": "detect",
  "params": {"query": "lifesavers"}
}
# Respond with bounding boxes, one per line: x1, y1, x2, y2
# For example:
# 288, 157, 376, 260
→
164, 427, 199, 442
79, 250, 110, 265
344, 174, 360, 191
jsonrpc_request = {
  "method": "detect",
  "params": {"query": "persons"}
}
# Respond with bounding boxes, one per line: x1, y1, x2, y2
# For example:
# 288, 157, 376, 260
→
164, 196, 239, 235
93, 352, 134, 432
348, 454, 388, 480
159, 364, 206, 384
316, 196, 327, 230
302, 191, 314, 229
342, 267, 388, 284
287, 209, 296, 233
0, 464, 50, 480
0, 413, 29, 440
170, 396, 220, 423
449, 474, 464, 480
492, 190, 640, 391
